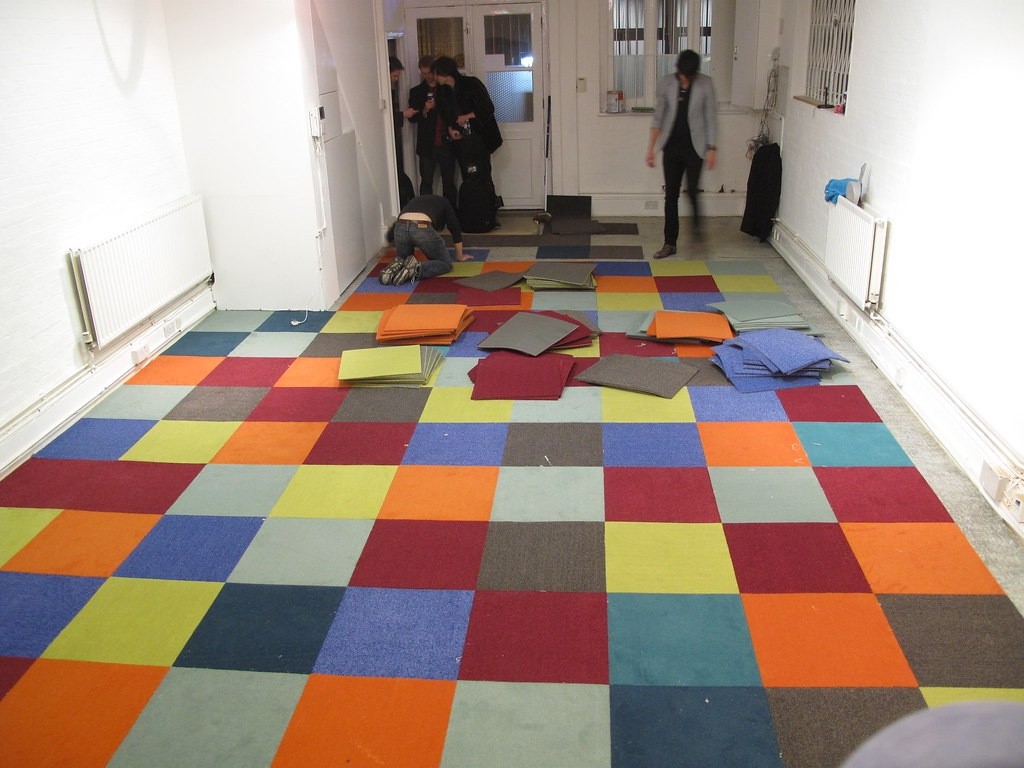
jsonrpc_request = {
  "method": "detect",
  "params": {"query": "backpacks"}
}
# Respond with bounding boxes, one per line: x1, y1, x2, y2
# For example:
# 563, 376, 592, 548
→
457, 171, 496, 233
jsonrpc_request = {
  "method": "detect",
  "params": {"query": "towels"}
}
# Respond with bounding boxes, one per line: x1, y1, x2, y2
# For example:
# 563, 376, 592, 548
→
823, 177, 863, 206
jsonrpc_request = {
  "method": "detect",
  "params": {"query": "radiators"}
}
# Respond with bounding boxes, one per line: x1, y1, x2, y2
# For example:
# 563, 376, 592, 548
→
824, 191, 888, 311
67, 194, 213, 351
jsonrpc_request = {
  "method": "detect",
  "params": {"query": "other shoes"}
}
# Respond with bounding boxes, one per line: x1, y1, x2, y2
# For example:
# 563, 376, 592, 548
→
653, 244, 678, 259
494, 223, 502, 229
691, 227, 703, 243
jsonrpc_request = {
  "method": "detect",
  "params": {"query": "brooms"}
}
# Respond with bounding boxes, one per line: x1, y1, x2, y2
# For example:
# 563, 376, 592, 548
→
532, 95, 552, 225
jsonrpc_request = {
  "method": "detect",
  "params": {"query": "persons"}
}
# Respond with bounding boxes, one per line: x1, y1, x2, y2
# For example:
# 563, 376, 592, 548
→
388, 55, 504, 230
646, 49, 717, 259
377, 194, 474, 287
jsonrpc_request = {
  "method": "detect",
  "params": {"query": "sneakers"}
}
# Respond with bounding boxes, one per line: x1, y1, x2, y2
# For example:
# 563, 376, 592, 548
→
393, 255, 420, 285
378, 256, 404, 285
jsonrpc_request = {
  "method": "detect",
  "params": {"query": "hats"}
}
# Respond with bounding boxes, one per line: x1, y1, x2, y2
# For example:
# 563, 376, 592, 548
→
676, 49, 700, 71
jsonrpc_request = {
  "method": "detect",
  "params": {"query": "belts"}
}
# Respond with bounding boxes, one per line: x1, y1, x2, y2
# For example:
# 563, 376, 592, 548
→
397, 219, 433, 227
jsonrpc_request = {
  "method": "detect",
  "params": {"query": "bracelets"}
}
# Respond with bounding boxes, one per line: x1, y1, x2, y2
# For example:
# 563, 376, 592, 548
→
707, 145, 716, 150
